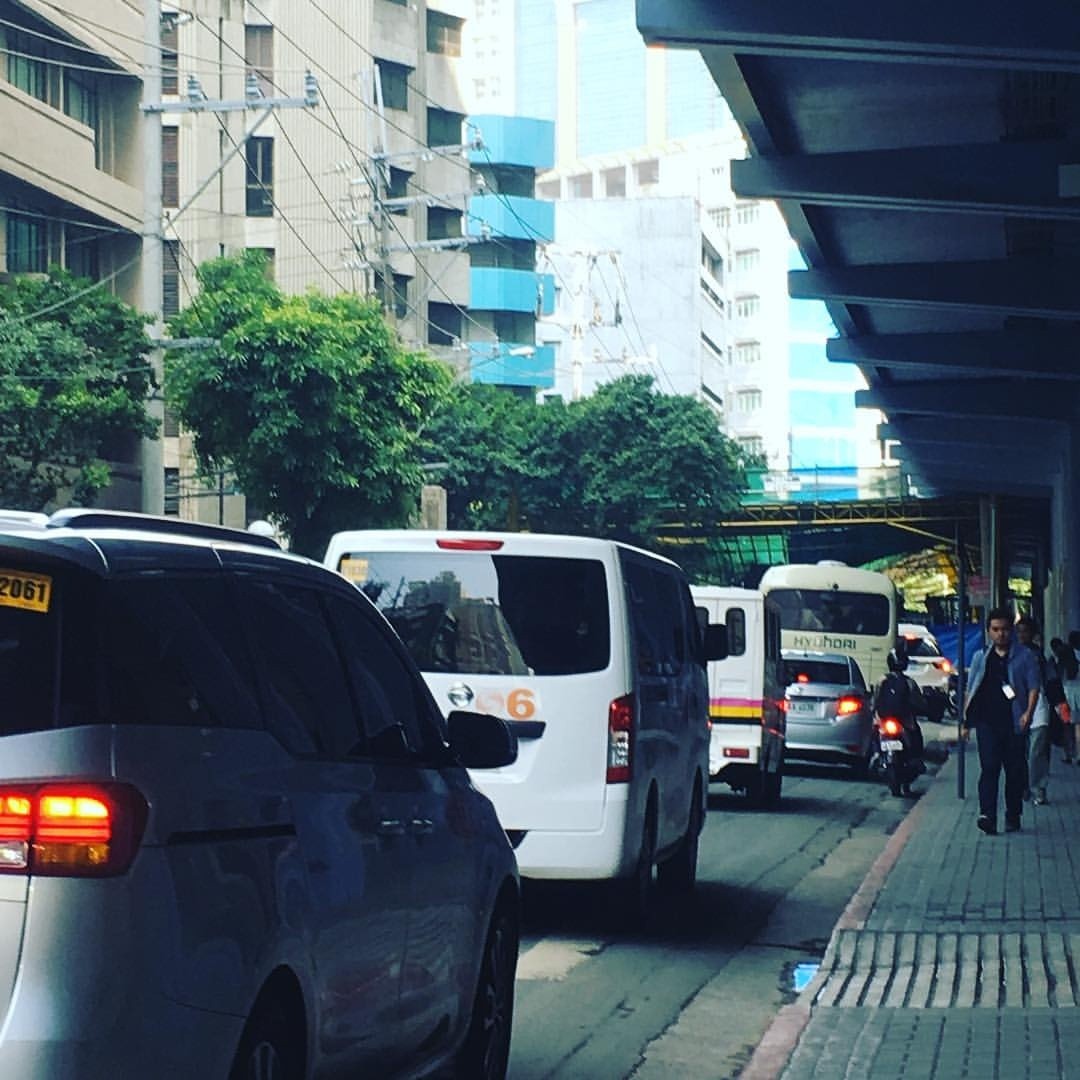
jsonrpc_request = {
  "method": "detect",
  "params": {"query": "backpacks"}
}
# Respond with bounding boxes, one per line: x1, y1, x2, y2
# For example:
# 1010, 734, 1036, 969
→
878, 673, 909, 717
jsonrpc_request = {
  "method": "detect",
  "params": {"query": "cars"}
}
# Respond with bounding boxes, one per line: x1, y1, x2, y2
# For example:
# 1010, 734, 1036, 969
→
781, 649, 873, 775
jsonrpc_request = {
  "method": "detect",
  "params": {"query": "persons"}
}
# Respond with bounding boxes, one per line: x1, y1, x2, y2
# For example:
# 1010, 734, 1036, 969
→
960, 605, 1080, 835
867, 647, 929, 776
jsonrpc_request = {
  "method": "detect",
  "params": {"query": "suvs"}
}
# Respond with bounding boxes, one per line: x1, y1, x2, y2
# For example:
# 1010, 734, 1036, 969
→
0, 508, 523, 1080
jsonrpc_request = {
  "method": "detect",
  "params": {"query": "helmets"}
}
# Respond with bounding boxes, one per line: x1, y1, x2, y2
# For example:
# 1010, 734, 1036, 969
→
886, 647, 908, 672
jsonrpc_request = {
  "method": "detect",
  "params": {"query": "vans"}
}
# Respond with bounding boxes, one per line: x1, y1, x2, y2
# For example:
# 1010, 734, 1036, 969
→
323, 530, 728, 933
691, 584, 795, 809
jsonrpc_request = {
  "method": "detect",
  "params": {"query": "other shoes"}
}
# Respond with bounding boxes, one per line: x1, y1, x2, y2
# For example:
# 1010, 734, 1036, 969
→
1032, 795, 1048, 806
978, 816, 997, 834
1005, 823, 1019, 832
1062, 757, 1073, 764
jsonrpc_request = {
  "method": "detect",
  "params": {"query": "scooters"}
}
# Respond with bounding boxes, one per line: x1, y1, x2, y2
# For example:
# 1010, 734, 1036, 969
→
875, 717, 927, 797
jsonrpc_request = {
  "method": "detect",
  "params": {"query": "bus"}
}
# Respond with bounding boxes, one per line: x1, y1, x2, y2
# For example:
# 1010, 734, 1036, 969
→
760, 560, 899, 693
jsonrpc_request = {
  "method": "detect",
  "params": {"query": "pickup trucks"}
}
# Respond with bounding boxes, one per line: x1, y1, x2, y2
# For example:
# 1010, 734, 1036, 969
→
897, 624, 951, 721
